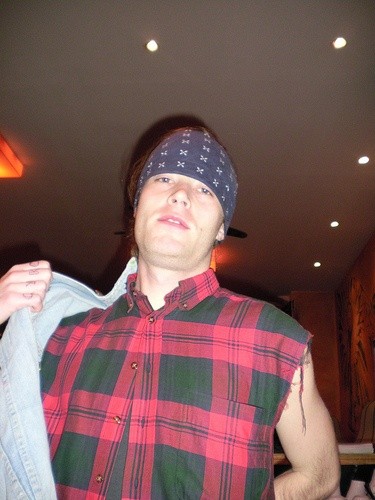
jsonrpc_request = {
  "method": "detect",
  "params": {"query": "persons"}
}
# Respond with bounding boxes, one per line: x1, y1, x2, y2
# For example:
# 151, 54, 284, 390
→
1, 123, 340, 500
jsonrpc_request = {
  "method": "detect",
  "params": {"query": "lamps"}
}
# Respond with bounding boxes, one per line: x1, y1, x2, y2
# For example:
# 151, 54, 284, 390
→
214, 227, 256, 264
0, 135, 24, 179
